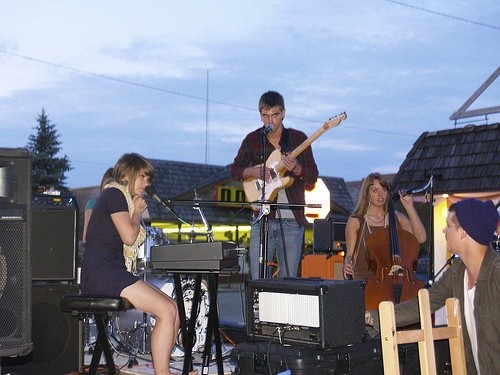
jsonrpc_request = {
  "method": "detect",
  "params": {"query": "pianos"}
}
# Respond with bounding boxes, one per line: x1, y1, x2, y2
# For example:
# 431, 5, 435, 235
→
150, 238, 239, 270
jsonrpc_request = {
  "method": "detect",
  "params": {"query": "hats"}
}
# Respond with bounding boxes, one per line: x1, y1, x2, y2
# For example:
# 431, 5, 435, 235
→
454, 198, 500, 246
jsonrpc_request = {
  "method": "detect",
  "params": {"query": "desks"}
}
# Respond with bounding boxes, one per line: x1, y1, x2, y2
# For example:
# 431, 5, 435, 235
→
166, 265, 241, 375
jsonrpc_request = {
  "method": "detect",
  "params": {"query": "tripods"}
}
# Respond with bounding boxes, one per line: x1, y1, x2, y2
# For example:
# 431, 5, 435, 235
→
112, 219, 158, 354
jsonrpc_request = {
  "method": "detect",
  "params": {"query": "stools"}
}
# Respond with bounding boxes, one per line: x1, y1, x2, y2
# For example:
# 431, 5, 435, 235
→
61, 296, 136, 375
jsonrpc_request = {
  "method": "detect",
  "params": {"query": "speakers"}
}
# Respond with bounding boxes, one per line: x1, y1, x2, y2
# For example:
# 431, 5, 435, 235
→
0, 147, 83, 375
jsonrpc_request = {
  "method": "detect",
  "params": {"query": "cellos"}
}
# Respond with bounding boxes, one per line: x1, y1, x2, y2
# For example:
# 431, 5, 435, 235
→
363, 180, 424, 312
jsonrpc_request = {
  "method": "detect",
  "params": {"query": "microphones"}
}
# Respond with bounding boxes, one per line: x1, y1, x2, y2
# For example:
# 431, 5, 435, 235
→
261, 124, 274, 135
146, 186, 180, 219
393, 189, 413, 201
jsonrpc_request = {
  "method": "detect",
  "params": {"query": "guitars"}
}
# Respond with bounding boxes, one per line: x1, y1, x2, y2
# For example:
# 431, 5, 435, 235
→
241, 110, 348, 211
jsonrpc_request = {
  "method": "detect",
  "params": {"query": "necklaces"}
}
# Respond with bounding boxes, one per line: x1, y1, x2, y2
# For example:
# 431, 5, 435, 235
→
367, 213, 386, 223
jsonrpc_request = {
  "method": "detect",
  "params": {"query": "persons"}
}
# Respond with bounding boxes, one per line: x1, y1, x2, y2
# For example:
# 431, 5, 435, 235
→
342, 171, 427, 331
81, 152, 197, 375
231, 90, 319, 280
365, 198, 500, 375
